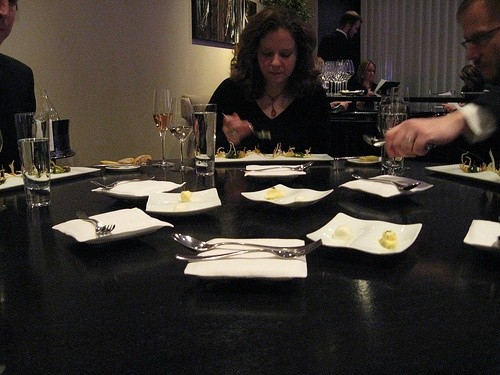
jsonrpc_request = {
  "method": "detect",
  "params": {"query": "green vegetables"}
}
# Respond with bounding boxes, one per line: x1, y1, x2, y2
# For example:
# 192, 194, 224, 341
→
292, 151, 305, 158
37, 166, 71, 175
226, 150, 240, 158
467, 162, 479, 173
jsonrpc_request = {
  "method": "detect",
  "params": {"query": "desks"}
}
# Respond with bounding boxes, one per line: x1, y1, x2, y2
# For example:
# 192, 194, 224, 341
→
326, 94, 464, 150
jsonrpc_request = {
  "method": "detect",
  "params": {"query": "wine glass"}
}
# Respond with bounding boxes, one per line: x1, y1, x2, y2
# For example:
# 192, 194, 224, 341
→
321, 60, 355, 96
153, 87, 194, 173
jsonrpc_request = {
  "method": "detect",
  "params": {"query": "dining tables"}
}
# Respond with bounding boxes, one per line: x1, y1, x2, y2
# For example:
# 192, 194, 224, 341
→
0, 148, 500, 375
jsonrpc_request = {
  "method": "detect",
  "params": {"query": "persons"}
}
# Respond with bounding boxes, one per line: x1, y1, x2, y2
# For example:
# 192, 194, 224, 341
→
385, 0, 500, 158
199, 6, 337, 155
0, 0, 36, 173
318, 11, 379, 94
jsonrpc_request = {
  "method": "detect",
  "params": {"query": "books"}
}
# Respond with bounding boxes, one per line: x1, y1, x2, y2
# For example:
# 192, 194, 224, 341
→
374, 78, 400, 95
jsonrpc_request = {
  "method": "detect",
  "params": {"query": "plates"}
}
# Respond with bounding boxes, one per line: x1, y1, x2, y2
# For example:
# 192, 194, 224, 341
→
50, 207, 174, 243
91, 179, 187, 200
305, 211, 422, 256
147, 187, 223, 218
241, 165, 334, 210
342, 156, 382, 165
338, 90, 364, 96
425, 164, 500, 183
340, 175, 434, 199
0, 166, 102, 190
108, 164, 139, 169
184, 239, 307, 280
196, 153, 335, 162
464, 220, 500, 251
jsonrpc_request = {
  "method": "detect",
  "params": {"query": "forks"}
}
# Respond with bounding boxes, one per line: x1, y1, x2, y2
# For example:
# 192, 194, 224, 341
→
352, 174, 421, 191
244, 160, 315, 171
89, 175, 156, 190
175, 239, 322, 261
74, 208, 115, 235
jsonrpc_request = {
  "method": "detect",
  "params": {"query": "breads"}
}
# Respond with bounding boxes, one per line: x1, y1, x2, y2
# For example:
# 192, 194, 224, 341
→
120, 154, 152, 165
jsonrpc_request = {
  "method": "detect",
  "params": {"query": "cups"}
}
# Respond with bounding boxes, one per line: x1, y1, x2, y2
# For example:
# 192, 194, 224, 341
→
193, 104, 217, 178
15, 112, 52, 207
378, 86, 407, 168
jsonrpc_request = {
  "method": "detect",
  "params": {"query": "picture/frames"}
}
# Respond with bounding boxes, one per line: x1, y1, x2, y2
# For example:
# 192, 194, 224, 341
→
192, 0, 257, 49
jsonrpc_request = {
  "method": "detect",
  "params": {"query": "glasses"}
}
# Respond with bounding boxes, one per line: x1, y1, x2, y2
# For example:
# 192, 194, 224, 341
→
459, 26, 500, 49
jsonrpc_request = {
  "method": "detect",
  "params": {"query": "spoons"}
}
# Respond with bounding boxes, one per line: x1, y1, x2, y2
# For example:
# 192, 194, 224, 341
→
170, 233, 306, 251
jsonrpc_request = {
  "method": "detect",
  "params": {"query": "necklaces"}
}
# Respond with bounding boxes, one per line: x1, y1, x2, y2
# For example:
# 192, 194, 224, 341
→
263, 87, 288, 115
